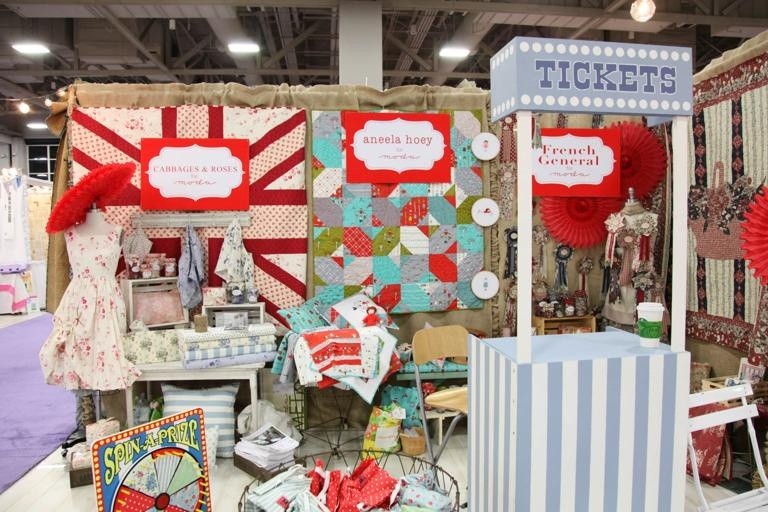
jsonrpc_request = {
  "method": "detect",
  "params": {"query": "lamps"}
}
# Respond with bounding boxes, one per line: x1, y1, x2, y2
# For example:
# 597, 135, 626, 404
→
628, 0, 658, 23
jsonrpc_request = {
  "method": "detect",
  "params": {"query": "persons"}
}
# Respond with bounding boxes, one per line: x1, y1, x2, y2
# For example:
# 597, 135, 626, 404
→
360, 306, 383, 327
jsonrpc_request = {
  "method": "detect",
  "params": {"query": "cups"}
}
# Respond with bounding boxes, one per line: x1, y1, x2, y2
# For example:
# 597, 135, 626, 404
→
636, 303, 664, 347
194, 315, 209, 332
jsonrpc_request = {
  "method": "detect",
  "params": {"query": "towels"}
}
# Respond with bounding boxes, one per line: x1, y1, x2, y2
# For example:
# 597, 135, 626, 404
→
176, 222, 206, 309
214, 220, 258, 303
178, 323, 276, 369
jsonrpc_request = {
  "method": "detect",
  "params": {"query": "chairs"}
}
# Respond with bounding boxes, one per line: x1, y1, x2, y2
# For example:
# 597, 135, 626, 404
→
411, 325, 472, 487
681, 380, 768, 512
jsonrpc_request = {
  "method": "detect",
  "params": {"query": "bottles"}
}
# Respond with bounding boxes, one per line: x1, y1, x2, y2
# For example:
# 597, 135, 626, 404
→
158, 252, 166, 275
147, 256, 160, 278
575, 290, 587, 317
565, 299, 575, 317
555, 304, 565, 318
128, 257, 139, 278
165, 258, 177, 276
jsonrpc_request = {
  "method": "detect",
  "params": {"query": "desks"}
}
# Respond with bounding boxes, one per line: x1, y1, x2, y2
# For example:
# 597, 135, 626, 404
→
288, 333, 394, 456
122, 361, 269, 451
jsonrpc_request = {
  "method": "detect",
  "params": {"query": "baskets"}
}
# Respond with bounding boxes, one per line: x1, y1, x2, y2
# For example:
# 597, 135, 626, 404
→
239, 448, 459, 512
701, 375, 768, 410
399, 427, 426, 455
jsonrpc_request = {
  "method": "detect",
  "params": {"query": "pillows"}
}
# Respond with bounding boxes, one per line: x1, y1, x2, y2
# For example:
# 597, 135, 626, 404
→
313, 292, 399, 331
159, 383, 240, 458
276, 297, 325, 334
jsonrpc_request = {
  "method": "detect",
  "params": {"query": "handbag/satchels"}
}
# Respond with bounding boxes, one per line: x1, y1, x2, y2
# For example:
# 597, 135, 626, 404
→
688, 160, 752, 261
